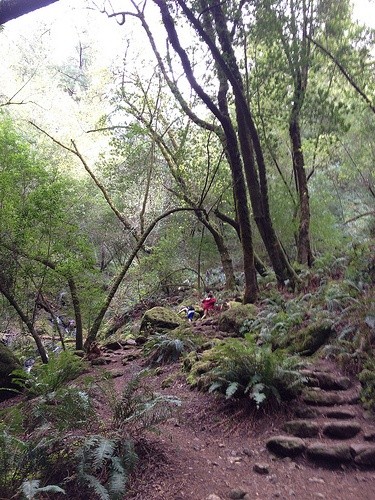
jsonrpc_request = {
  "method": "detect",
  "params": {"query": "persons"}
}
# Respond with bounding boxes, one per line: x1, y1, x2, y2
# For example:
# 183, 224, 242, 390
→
200, 293, 216, 318
177, 305, 194, 322
